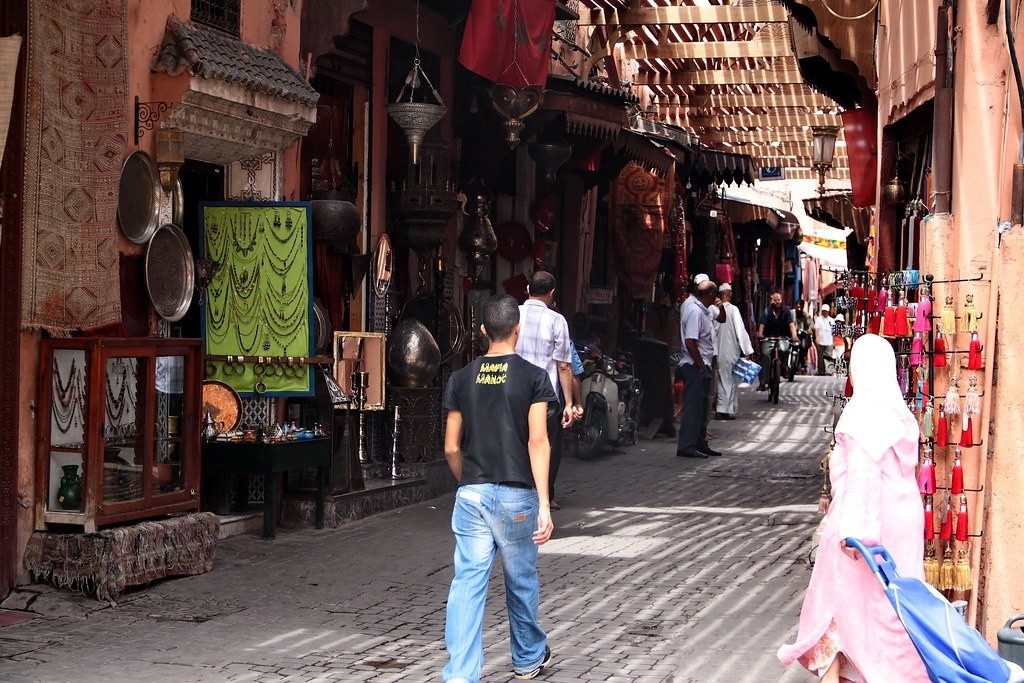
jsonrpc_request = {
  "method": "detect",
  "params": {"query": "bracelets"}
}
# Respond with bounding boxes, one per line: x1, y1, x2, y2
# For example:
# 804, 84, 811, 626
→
106, 421, 137, 437
205, 363, 307, 378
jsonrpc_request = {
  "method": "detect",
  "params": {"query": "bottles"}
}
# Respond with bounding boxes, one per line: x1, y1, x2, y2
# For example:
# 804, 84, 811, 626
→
243, 421, 324, 444
58, 464, 81, 510
103, 447, 134, 503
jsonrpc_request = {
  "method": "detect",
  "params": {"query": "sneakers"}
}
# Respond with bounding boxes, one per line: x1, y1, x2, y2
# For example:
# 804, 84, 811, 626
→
514, 645, 552, 680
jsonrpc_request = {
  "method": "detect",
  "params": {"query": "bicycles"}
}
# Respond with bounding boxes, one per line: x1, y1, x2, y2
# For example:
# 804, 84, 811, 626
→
761, 335, 799, 405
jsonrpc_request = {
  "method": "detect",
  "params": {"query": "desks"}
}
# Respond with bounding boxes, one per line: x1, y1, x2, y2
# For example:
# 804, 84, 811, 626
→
203, 434, 332, 540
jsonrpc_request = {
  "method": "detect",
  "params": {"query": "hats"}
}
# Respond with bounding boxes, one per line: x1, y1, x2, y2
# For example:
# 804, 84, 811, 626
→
719, 282, 732, 292
834, 314, 845, 322
694, 274, 709, 284
822, 304, 830, 312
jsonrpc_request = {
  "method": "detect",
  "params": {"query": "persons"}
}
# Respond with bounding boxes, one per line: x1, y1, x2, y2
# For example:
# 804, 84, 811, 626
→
712, 283, 754, 422
777, 334, 932, 683
514, 270, 573, 511
814, 303, 836, 375
757, 292, 796, 392
441, 292, 559, 683
674, 274, 726, 458
549, 298, 585, 422
831, 314, 851, 361
789, 299, 814, 372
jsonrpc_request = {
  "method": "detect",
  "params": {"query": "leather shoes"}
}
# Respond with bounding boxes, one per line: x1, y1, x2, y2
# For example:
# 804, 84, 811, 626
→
676, 449, 708, 459
696, 446, 722, 456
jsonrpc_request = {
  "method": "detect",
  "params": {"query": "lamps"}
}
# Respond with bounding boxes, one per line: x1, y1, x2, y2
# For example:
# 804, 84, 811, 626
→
156, 128, 184, 197
808, 124, 852, 193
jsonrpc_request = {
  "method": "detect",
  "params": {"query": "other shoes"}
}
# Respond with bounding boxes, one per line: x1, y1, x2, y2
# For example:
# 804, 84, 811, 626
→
550, 500, 560, 511
715, 412, 737, 420
756, 384, 766, 391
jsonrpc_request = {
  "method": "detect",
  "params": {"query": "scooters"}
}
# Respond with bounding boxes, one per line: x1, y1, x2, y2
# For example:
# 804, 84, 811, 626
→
780, 331, 812, 383
575, 342, 648, 460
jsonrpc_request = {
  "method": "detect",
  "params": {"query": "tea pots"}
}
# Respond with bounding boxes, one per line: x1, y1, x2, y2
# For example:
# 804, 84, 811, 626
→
202, 408, 225, 441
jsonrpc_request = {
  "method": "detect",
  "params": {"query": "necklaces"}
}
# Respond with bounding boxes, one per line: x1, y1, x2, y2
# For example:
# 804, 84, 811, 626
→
52, 354, 137, 433
487, 351, 508, 357
203, 211, 306, 358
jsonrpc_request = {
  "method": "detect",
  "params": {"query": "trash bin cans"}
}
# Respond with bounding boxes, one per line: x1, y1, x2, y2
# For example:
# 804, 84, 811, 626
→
996, 614, 1024, 671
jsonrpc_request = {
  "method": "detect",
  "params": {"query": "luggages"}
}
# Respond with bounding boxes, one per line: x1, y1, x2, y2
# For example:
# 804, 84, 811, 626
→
845, 537, 1024, 683
